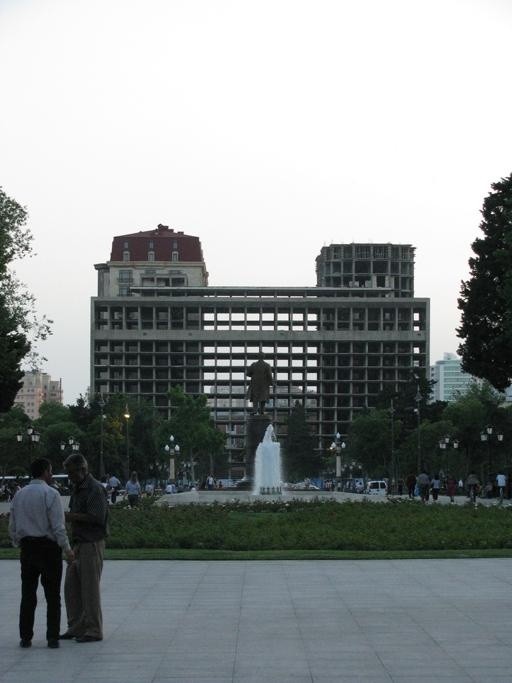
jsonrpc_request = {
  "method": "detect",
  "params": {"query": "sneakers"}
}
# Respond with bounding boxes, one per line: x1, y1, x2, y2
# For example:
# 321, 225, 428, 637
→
47, 638, 61, 648
19, 636, 34, 648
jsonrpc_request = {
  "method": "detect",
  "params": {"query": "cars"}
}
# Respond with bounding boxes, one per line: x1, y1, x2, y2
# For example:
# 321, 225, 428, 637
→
280, 476, 365, 493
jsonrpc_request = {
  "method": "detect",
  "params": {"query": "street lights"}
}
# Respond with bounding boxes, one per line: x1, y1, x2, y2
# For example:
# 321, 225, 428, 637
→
99, 389, 106, 480
60, 435, 81, 490
412, 408, 422, 475
17, 424, 41, 482
330, 431, 347, 491
165, 435, 180, 487
124, 401, 130, 483
439, 431, 460, 491
479, 421, 505, 496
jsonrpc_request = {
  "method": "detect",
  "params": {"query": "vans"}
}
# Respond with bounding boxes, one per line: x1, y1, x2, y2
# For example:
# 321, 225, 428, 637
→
365, 480, 389, 497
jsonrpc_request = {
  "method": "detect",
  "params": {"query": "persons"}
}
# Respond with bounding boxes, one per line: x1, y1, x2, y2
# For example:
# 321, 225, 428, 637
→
1, 474, 61, 501
60, 454, 108, 644
97, 471, 178, 508
304, 476, 364, 494
247, 353, 277, 414
383, 469, 507, 504
10, 459, 76, 648
205, 472, 223, 490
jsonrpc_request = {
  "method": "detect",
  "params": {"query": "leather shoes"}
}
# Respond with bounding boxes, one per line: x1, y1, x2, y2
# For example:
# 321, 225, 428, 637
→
74, 633, 105, 644
57, 630, 82, 639
